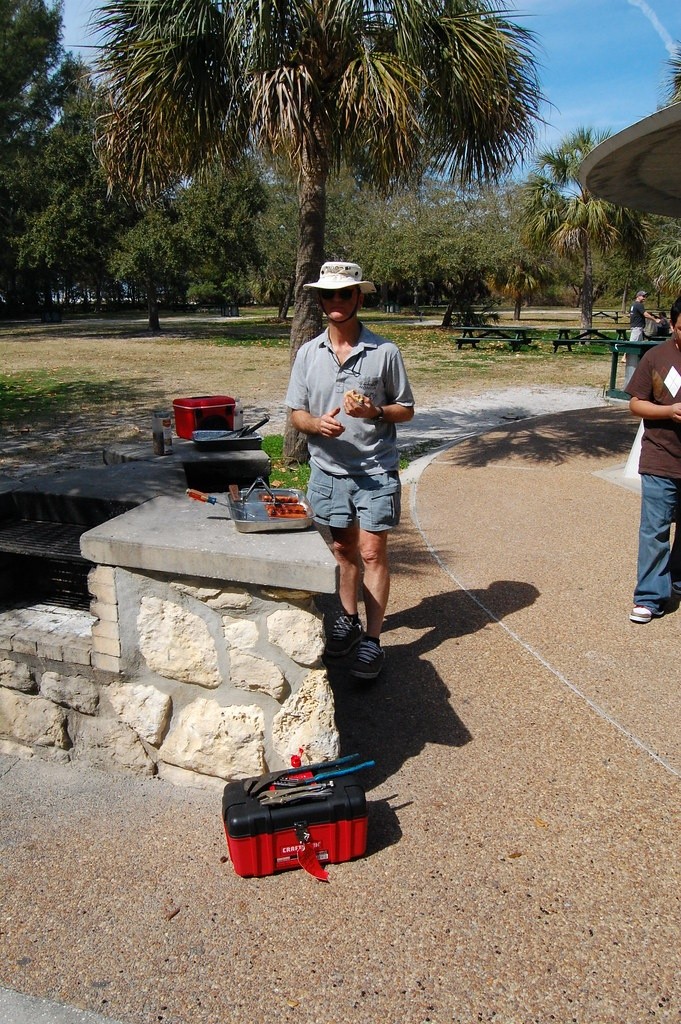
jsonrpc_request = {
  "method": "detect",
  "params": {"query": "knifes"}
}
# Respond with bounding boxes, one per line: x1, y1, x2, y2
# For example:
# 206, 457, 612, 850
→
228, 484, 246, 521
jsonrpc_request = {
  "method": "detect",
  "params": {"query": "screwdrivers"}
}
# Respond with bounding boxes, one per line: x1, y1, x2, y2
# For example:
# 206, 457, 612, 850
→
185, 487, 256, 519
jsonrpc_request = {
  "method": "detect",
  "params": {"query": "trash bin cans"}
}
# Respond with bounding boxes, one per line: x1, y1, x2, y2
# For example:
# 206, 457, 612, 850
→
231, 301, 239, 316
221, 301, 229, 317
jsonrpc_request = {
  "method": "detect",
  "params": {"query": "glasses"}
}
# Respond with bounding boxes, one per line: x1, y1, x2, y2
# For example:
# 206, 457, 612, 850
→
322, 287, 357, 299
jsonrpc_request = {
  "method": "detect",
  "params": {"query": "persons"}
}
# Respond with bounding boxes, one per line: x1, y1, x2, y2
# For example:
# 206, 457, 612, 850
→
658, 312, 670, 336
624, 293, 680, 626
644, 311, 660, 337
620, 290, 662, 365
288, 263, 419, 675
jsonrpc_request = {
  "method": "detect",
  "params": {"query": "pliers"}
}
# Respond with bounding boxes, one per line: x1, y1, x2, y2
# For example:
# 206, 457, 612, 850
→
257, 783, 332, 806
243, 753, 377, 796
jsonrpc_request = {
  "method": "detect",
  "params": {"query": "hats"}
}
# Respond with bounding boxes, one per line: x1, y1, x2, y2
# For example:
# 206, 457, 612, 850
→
303, 262, 376, 294
636, 291, 647, 296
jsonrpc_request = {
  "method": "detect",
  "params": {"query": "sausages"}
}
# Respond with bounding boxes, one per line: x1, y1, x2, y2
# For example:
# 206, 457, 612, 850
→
260, 495, 308, 520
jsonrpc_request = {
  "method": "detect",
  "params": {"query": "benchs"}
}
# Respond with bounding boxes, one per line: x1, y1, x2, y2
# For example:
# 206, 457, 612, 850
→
457, 337, 519, 351
485, 336, 542, 347
551, 339, 623, 353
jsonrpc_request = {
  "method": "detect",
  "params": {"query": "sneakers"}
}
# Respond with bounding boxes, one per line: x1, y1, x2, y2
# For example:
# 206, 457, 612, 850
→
630, 581, 681, 623
327, 612, 386, 681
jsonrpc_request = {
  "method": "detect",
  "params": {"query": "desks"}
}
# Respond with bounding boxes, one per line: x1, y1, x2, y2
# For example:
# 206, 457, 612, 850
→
548, 327, 631, 340
602, 341, 670, 402
452, 326, 527, 348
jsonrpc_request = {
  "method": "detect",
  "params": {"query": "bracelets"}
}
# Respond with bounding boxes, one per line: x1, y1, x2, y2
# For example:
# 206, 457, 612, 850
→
376, 406, 384, 421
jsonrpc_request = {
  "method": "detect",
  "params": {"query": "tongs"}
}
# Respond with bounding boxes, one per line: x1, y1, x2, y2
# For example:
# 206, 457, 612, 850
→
241, 476, 282, 507
209, 425, 249, 439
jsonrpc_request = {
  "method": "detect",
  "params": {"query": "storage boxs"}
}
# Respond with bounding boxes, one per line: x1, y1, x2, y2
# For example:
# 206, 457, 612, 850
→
171, 395, 236, 438
222, 767, 369, 877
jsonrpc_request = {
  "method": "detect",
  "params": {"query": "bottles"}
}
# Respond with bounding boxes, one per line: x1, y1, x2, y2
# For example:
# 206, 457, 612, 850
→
234, 398, 244, 431
152, 412, 174, 456
